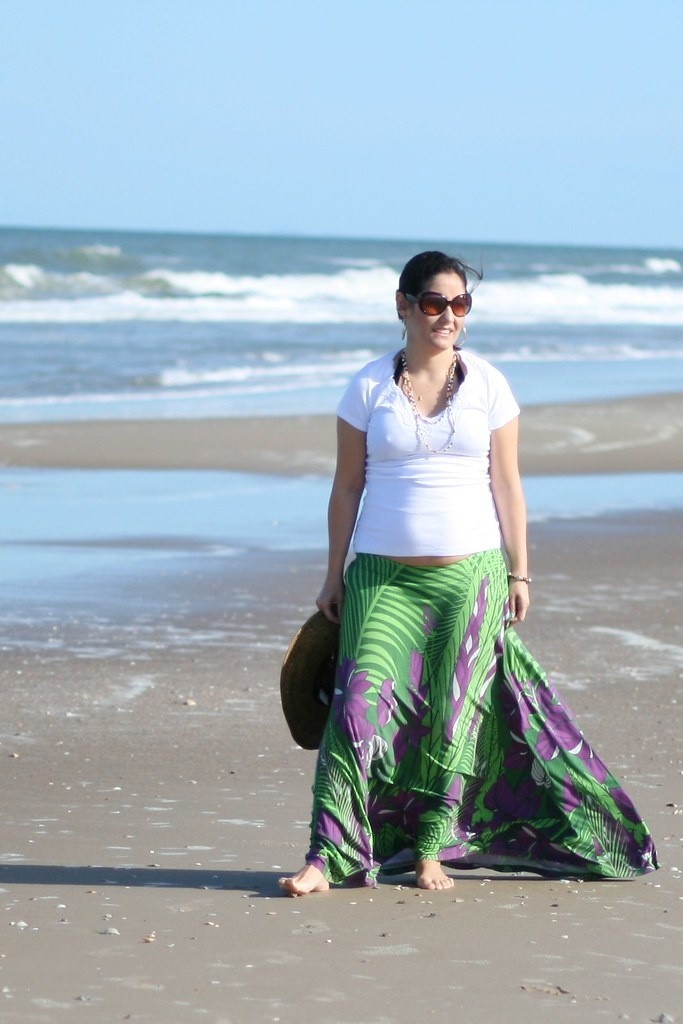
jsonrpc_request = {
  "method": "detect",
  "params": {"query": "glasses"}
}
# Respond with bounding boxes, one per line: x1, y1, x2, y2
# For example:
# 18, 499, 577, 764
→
404, 292, 472, 316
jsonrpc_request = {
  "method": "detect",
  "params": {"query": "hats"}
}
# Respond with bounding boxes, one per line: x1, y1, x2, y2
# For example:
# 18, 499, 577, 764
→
279, 604, 339, 751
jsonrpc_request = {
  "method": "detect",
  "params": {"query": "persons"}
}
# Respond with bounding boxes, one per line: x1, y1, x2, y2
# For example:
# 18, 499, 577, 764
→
276, 247, 532, 904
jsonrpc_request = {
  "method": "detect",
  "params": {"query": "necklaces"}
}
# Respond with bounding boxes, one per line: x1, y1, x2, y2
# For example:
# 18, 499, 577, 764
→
407, 366, 449, 400
400, 348, 458, 455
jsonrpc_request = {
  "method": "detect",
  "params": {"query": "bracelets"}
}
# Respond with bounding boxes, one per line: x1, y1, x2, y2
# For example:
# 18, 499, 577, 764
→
506, 571, 532, 587
